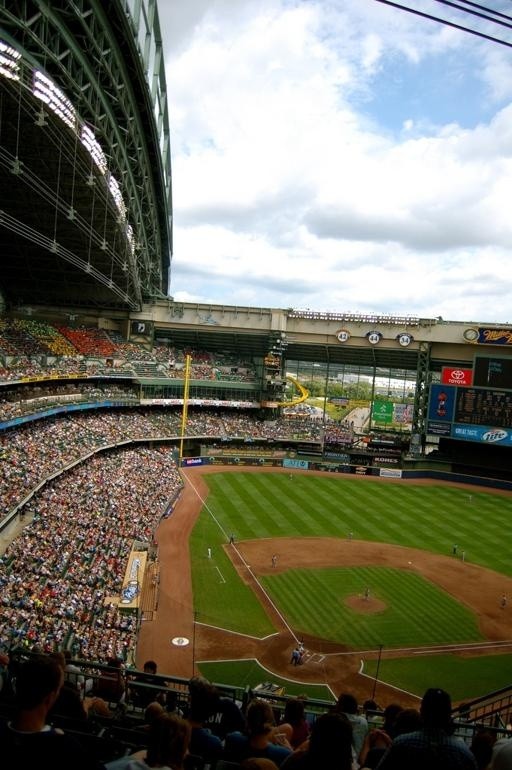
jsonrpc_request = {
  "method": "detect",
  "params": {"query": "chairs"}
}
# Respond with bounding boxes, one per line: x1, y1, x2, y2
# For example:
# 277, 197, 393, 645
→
1, 443, 172, 678
0, 316, 281, 440
59, 709, 245, 770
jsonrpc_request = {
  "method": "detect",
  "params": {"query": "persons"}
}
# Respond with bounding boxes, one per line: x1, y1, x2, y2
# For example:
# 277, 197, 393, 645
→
452, 543, 458, 558
227, 532, 238, 546
207, 545, 212, 560
364, 587, 370, 601
500, 594, 508, 610
297, 644, 305, 664
270, 553, 278, 568
289, 647, 300, 667
184, 345, 355, 467
0, 655, 108, 769
